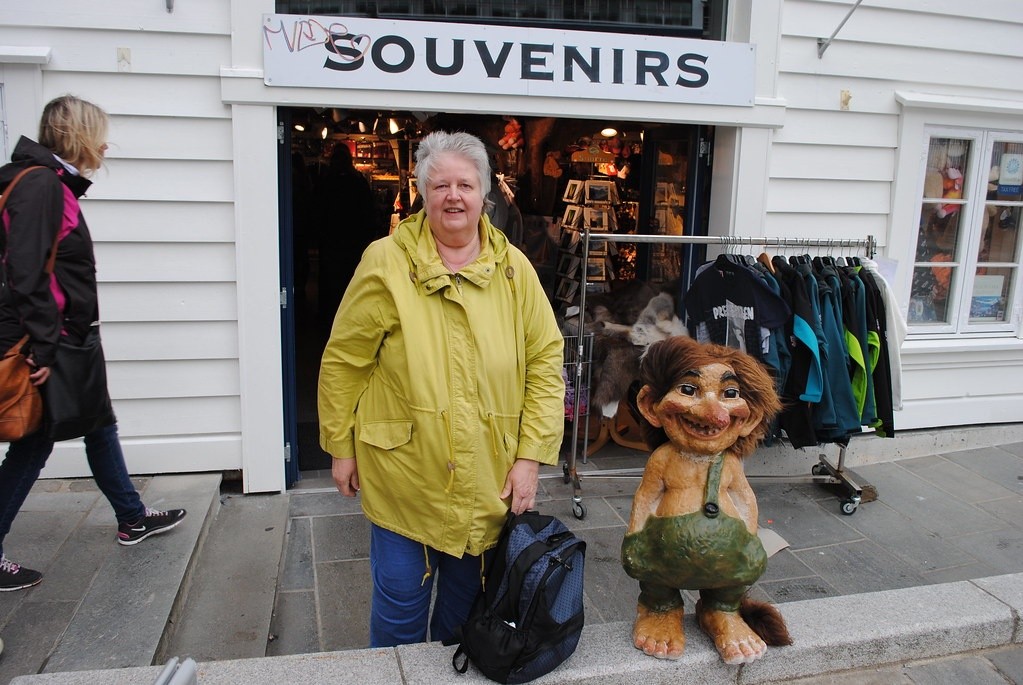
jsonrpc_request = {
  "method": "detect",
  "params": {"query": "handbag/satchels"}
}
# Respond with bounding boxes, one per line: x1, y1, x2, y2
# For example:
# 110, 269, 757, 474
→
0, 335, 43, 441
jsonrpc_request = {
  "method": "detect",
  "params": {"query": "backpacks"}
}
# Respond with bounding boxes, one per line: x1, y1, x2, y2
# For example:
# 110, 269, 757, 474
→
442, 510, 588, 685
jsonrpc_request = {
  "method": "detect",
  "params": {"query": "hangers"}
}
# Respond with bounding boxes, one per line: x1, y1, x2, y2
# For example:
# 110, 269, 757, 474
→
712, 235, 867, 276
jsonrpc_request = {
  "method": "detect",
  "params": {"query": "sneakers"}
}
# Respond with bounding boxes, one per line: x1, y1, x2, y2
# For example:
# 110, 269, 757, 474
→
0, 552, 43, 592
116, 507, 187, 545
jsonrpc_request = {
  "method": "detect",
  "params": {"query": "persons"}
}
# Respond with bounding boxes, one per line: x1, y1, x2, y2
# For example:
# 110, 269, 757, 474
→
620, 340, 782, 663
319, 131, 565, 647
0, 98, 188, 593
303, 140, 525, 329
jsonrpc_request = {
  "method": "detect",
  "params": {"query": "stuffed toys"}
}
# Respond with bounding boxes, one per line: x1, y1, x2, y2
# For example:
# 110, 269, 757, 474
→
914, 138, 1000, 295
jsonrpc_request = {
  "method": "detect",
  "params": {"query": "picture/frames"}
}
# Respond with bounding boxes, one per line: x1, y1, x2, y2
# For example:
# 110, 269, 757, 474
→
554, 178, 622, 303
648, 181, 668, 283
